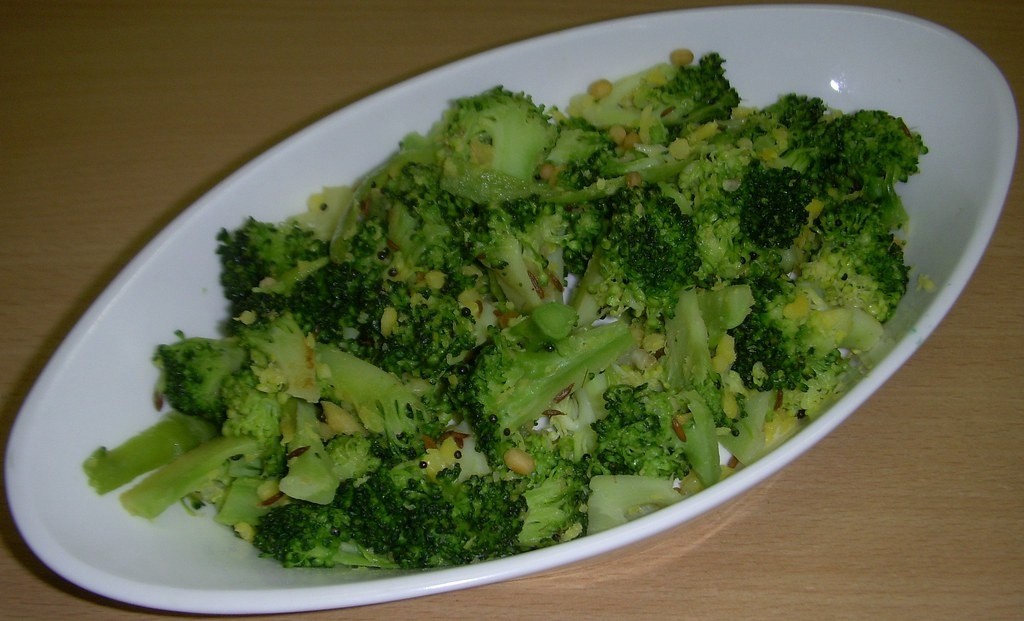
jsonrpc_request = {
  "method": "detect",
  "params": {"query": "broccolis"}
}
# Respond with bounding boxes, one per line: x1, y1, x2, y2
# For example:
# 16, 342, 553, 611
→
77, 49, 929, 567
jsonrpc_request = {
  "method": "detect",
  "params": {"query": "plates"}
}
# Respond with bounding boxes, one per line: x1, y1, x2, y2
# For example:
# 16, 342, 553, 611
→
5, 5, 1017, 615
